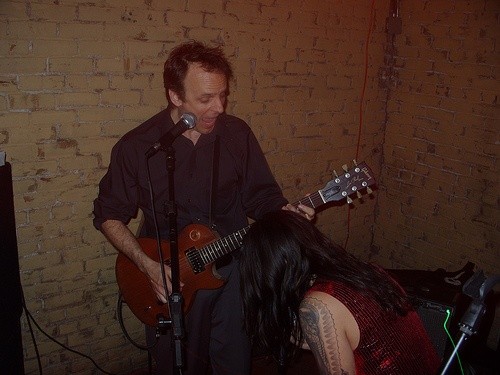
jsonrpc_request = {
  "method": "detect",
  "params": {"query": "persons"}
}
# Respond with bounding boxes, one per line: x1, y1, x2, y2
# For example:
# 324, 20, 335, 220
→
240, 209, 441, 375
92, 39, 316, 375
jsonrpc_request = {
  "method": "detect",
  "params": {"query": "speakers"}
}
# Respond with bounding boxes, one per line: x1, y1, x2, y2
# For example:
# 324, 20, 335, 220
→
402, 289, 456, 365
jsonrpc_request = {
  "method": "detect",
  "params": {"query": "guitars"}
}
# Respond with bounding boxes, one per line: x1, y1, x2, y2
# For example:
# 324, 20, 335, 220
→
114, 158, 376, 332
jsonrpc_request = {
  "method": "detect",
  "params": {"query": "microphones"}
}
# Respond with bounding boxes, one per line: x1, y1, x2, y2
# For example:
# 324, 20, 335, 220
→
147, 114, 196, 158
